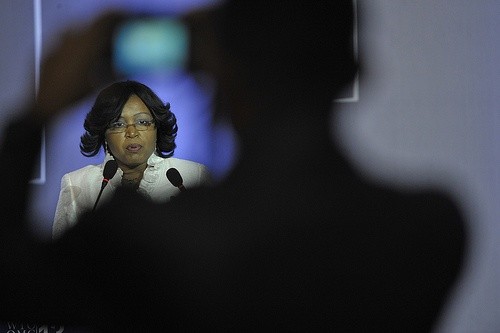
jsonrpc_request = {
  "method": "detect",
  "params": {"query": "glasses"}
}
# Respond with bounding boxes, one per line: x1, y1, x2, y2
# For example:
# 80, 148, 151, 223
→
106, 119, 156, 131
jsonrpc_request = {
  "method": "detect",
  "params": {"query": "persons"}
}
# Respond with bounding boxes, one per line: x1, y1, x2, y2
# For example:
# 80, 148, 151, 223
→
51, 79, 216, 239
1, 0, 472, 333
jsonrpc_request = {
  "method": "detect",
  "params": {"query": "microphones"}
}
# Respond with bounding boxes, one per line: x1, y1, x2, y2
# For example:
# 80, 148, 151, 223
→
166, 168, 185, 191
93, 160, 118, 208
